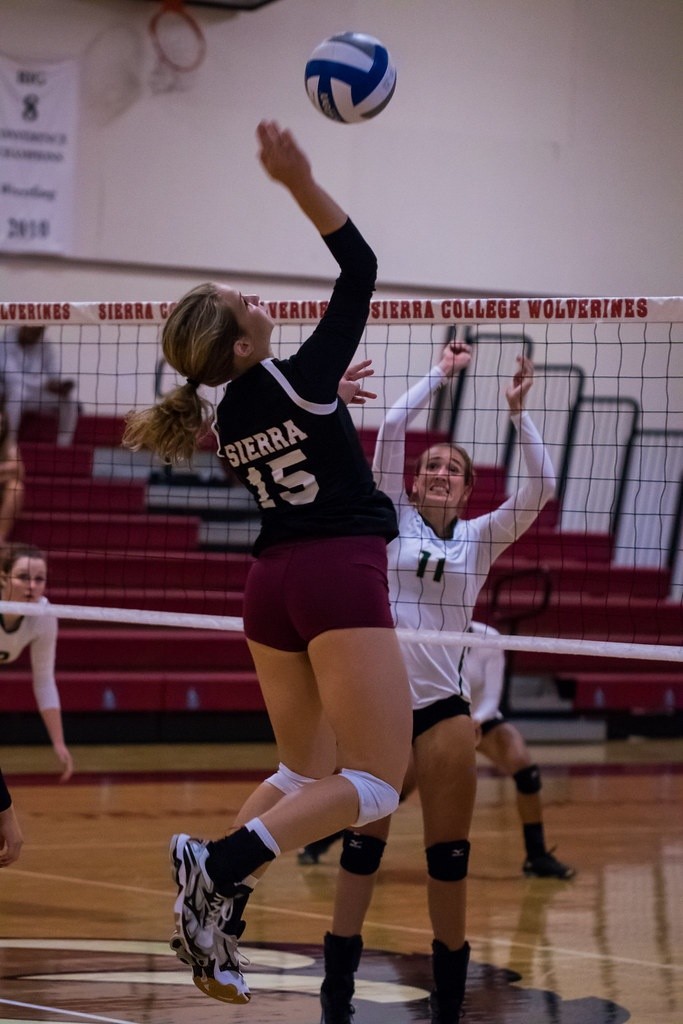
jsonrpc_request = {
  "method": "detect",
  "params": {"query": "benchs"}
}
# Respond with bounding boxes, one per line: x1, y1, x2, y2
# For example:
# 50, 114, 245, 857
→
0, 407, 683, 745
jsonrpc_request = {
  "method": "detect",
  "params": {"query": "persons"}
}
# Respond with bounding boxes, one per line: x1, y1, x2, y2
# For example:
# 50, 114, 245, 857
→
123, 118, 410, 1006
0, 321, 80, 449
293, 618, 572, 880
1, 464, 79, 874
317, 344, 556, 1023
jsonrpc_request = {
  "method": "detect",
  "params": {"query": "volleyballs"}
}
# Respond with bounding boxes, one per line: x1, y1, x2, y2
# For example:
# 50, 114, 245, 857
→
301, 31, 401, 127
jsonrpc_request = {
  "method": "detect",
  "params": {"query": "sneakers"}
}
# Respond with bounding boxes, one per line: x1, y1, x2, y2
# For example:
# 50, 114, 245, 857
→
170, 919, 253, 1005
321, 978, 354, 1024
430, 986, 465, 1023
298, 836, 331, 863
169, 833, 233, 968
524, 846, 574, 881
